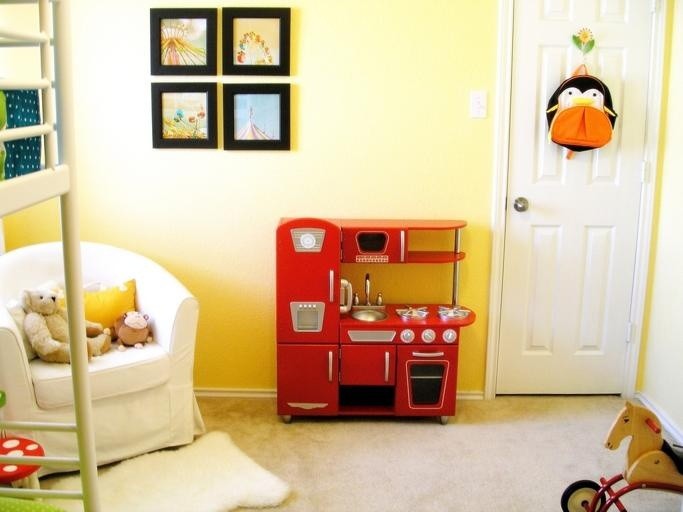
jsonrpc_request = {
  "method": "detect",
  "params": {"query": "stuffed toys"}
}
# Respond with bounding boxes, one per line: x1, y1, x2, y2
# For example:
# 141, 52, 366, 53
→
19, 283, 112, 364
544, 71, 618, 161
112, 309, 153, 352
